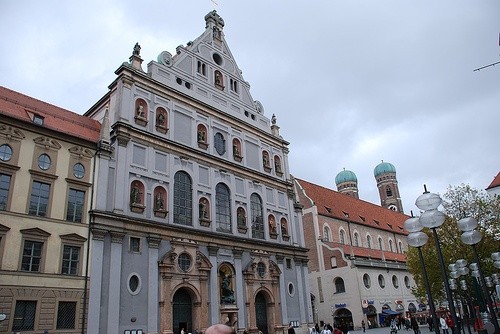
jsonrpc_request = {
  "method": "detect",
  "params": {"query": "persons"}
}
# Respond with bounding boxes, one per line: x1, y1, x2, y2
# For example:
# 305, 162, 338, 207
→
132, 183, 164, 208
409, 313, 428, 334
215, 72, 220, 84
262, 152, 281, 173
427, 313, 455, 334
463, 312, 468, 327
222, 270, 234, 299
181, 324, 236, 334
288, 324, 295, 334
473, 306, 483, 334
132, 43, 141, 56
361, 319, 372, 333
310, 321, 348, 334
237, 208, 246, 229
200, 200, 208, 218
268, 218, 286, 234
197, 130, 239, 154
390, 315, 411, 334
486, 320, 494, 334
137, 101, 166, 126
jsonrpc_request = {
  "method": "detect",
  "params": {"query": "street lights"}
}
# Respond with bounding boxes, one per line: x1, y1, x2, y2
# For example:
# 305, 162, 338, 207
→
415, 184, 461, 333
457, 211, 500, 334
442, 251, 500, 334
403, 209, 441, 334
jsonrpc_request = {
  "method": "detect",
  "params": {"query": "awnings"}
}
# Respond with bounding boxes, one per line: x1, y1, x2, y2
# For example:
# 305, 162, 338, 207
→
383, 309, 401, 315
